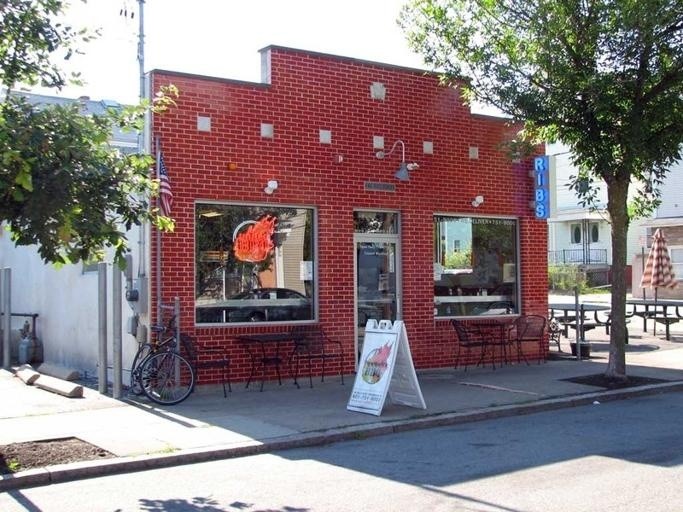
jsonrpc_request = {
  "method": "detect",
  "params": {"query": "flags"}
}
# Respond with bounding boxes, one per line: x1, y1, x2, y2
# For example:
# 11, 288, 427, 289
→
157, 148, 173, 219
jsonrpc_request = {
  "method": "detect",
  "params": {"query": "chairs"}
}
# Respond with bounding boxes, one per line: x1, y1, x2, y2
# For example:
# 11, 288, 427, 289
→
508, 315, 547, 365
451, 319, 496, 373
292, 325, 345, 388
181, 334, 232, 398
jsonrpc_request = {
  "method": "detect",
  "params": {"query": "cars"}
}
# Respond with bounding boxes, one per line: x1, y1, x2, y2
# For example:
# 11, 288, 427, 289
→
196, 287, 312, 324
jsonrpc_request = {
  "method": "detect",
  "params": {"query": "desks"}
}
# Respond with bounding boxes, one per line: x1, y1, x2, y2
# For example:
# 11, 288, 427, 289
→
626, 300, 683, 336
548, 303, 610, 338
235, 334, 301, 392
471, 322, 530, 369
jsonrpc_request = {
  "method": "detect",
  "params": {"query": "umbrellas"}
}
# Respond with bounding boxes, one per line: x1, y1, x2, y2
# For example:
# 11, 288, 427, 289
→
639, 228, 678, 337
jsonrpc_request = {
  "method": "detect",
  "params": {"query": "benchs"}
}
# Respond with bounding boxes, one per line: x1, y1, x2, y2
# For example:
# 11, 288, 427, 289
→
552, 311, 683, 344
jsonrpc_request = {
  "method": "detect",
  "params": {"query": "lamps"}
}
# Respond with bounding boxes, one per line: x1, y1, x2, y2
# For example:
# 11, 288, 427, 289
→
376, 140, 409, 181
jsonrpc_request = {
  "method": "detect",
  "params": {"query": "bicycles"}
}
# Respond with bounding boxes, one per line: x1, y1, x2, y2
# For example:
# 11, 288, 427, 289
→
130, 314, 195, 406
